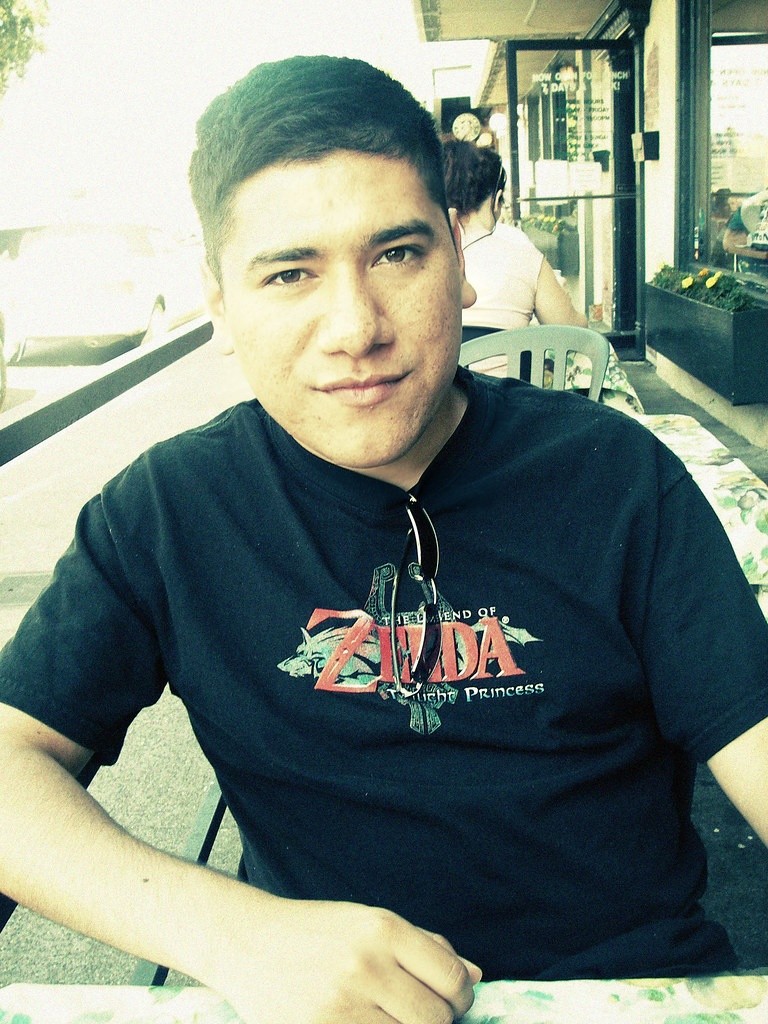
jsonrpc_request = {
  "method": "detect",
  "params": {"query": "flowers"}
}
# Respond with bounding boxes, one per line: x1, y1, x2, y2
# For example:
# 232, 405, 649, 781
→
649, 260, 758, 312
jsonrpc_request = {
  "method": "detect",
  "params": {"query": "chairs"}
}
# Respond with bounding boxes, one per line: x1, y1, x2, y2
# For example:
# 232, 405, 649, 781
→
458, 323, 610, 404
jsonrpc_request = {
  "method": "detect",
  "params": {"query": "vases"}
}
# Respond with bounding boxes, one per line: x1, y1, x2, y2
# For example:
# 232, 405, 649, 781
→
643, 280, 768, 406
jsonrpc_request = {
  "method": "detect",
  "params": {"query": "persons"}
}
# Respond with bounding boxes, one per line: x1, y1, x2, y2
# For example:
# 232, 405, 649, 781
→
710, 186, 767, 263
444, 134, 589, 344
1, 54, 768, 1024
